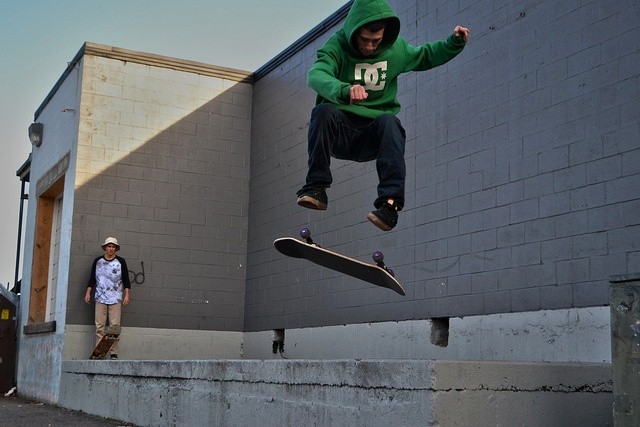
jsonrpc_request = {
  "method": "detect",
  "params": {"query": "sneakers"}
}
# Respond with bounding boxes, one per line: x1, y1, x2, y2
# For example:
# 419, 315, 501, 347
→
367, 198, 398, 231
111, 354, 117, 359
297, 187, 328, 210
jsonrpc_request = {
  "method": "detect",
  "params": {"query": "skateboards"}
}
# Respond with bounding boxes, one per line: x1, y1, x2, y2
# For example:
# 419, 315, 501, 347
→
89, 324, 121, 360
273, 228, 405, 296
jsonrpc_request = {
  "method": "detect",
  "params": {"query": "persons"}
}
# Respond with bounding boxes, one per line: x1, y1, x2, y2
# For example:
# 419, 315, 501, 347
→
84, 237, 131, 360
296, 1, 470, 231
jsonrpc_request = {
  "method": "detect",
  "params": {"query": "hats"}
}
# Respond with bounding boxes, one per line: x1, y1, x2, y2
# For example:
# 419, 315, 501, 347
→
101, 237, 120, 251
